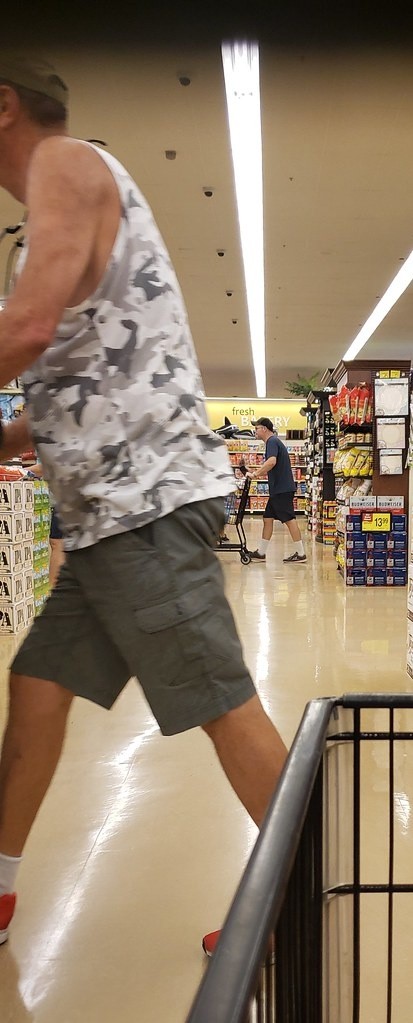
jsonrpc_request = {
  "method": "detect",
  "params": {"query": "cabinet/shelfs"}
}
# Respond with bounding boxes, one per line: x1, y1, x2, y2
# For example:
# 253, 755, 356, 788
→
298, 358, 409, 545
225, 439, 309, 511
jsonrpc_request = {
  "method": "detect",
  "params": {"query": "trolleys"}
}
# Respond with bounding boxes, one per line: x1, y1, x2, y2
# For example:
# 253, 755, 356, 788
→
214, 476, 253, 565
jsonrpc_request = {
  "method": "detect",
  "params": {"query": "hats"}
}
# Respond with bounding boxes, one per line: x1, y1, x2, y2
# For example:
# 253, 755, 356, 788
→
251, 417, 273, 433
0, 44, 69, 105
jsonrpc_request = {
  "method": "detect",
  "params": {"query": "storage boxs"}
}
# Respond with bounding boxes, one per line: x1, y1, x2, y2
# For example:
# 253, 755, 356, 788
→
334, 514, 407, 586
224, 439, 306, 511
0, 395, 51, 637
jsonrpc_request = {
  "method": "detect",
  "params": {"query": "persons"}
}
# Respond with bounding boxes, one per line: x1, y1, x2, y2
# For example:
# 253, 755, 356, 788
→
245, 417, 309, 563
0, 56, 292, 962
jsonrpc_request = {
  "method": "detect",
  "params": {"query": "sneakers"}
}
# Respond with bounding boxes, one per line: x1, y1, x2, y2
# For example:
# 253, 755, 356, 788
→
0, 892, 17, 944
283, 551, 308, 563
248, 549, 266, 562
202, 929, 277, 967
